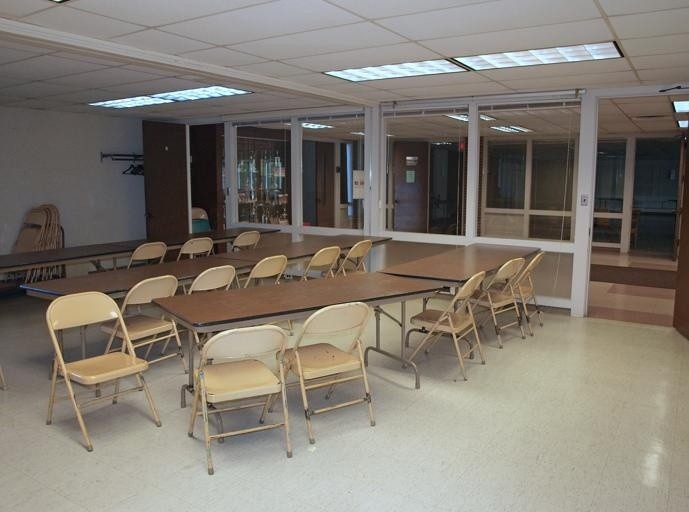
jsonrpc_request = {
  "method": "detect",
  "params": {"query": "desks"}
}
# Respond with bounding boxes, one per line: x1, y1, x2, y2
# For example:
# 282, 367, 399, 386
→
152, 271, 443, 443
377, 244, 540, 360
212, 234, 392, 262
26, 256, 256, 300
1, 243, 137, 288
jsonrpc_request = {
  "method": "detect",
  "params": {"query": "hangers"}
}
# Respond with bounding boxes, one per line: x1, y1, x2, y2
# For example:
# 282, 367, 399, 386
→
120, 154, 146, 177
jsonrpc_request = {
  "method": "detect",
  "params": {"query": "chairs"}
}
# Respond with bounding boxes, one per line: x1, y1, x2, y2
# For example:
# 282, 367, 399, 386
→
478, 251, 546, 337
228, 231, 261, 252
618, 206, 641, 250
403, 270, 485, 381
100, 275, 189, 375
188, 325, 294, 476
160, 265, 236, 357
268, 301, 375, 444
46, 291, 161, 452
243, 255, 287, 288
176, 237, 213, 262
300, 246, 341, 281
335, 240, 372, 277
470, 257, 527, 348
4, 204, 62, 285
127, 241, 167, 269
